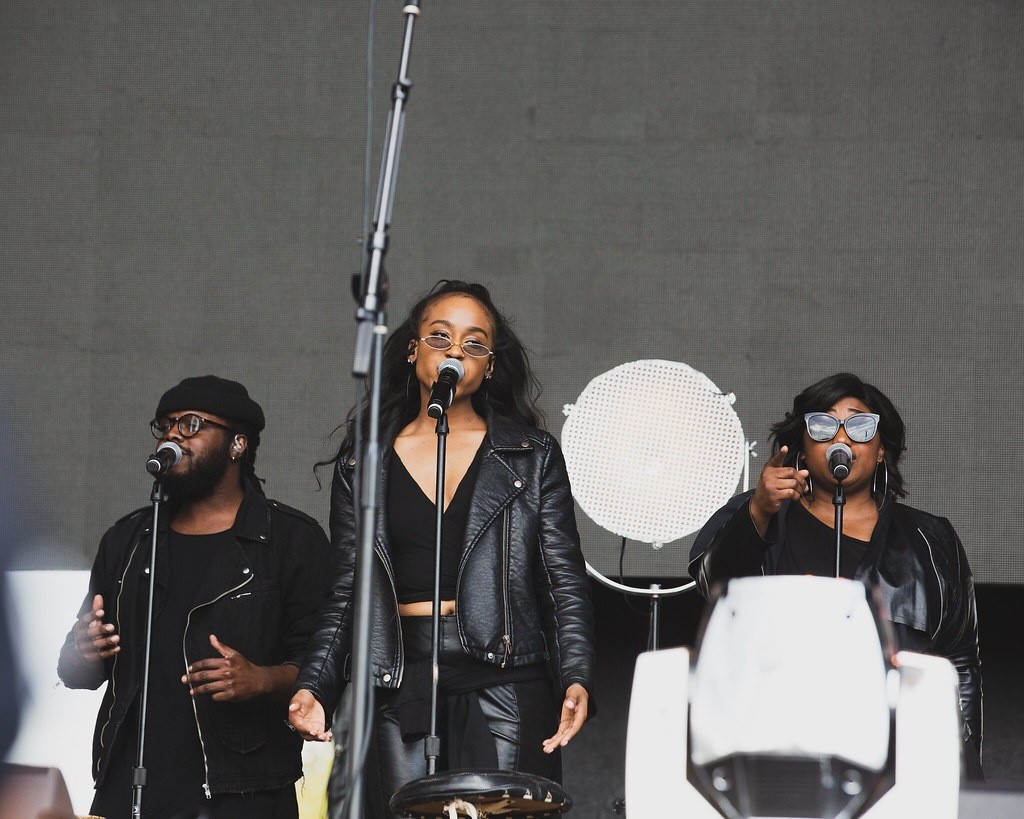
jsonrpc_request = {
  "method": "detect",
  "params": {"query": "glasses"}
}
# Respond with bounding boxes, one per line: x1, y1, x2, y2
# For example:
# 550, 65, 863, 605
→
150, 413, 234, 440
804, 412, 880, 443
418, 335, 494, 358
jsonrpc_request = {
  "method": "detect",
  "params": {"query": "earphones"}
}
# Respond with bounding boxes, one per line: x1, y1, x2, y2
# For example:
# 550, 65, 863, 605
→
233, 437, 242, 454
407, 345, 416, 356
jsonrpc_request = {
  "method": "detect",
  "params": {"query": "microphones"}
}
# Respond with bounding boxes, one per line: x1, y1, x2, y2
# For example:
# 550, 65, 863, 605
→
427, 358, 465, 419
826, 442, 853, 479
146, 442, 182, 474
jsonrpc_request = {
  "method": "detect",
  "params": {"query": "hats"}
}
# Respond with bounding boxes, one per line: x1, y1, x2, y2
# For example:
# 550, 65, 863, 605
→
155, 374, 265, 432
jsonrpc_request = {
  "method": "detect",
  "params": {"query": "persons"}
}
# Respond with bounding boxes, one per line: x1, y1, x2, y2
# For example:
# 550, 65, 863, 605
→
57, 375, 330, 819
687, 372, 986, 789
287, 278, 606, 819
